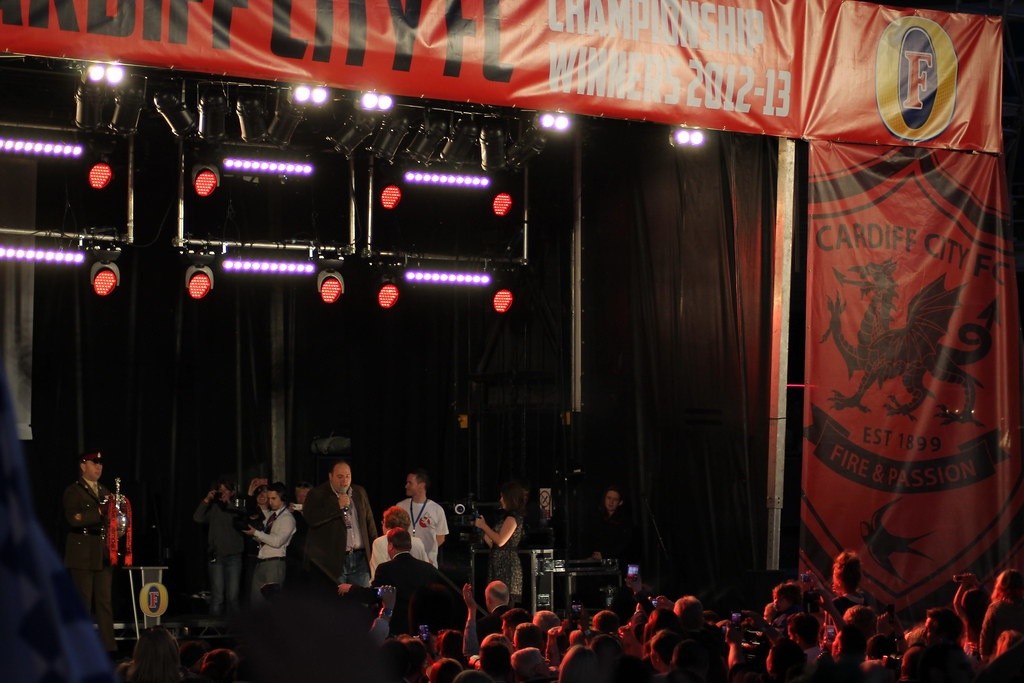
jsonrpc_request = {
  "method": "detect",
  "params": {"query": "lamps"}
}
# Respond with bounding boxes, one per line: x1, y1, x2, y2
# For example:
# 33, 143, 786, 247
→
72, 64, 567, 319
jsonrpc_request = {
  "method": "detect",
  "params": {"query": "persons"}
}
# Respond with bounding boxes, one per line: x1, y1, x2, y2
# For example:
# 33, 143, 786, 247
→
581, 488, 646, 604
62, 449, 116, 654
475, 481, 527, 606
395, 469, 449, 569
122, 553, 1024, 683
196, 477, 309, 613
369, 506, 429, 582
338, 528, 443, 638
303, 459, 376, 586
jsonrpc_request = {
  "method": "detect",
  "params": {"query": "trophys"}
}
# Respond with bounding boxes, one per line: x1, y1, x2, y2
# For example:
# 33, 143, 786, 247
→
98, 477, 127, 542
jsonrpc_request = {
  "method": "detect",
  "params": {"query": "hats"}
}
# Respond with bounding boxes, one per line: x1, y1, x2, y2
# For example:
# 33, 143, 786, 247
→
78, 447, 103, 464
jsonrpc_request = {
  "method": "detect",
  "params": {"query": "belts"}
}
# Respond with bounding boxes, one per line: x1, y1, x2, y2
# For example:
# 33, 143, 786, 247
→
257, 556, 286, 563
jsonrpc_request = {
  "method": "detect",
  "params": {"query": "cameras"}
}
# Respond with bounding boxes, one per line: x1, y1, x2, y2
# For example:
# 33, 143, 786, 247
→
801, 574, 812, 582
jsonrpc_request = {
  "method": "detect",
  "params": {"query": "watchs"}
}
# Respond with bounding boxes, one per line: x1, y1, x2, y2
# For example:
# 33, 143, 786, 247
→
379, 608, 393, 617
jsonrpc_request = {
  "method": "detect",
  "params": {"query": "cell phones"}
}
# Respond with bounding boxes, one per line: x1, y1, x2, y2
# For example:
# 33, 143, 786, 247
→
570, 600, 583, 622
626, 564, 639, 588
887, 604, 895, 624
825, 627, 836, 642
214, 492, 222, 498
730, 611, 742, 631
419, 624, 430, 641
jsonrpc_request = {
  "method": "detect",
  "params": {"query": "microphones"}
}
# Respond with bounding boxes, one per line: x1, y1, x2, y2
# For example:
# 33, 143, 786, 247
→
341, 488, 349, 511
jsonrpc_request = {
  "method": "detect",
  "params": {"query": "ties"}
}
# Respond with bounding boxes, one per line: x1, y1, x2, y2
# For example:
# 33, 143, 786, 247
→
260, 512, 276, 547
93, 482, 97, 495
